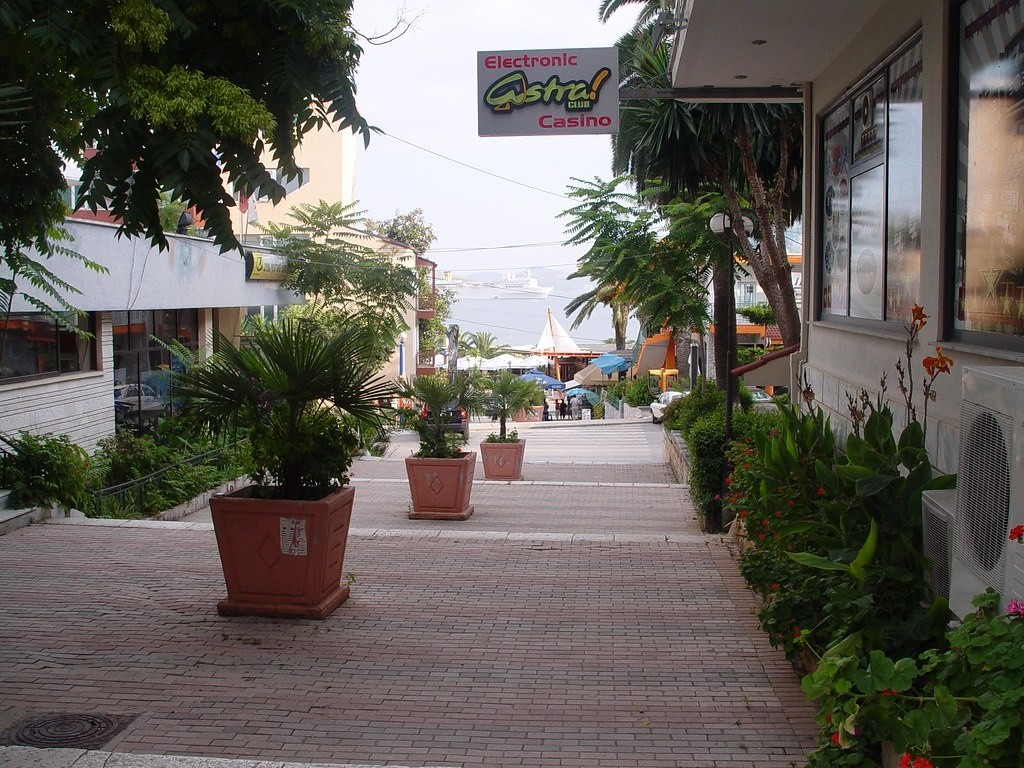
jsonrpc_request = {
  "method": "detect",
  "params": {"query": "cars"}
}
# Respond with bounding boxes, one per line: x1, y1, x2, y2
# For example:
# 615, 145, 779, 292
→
650, 392, 686, 424
419, 396, 470, 441
486, 402, 511, 418
115, 384, 175, 421
733, 385, 788, 413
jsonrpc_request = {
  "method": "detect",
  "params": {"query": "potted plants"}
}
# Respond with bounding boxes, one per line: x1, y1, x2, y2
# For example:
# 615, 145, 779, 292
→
161, 314, 548, 620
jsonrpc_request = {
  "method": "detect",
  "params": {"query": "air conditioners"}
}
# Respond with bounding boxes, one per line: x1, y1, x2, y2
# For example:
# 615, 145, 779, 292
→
922, 490, 957, 598
948, 364, 1024, 623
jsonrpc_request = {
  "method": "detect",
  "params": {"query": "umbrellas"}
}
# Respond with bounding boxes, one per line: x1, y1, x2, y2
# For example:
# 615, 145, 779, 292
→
444, 353, 560, 378
509, 368, 566, 391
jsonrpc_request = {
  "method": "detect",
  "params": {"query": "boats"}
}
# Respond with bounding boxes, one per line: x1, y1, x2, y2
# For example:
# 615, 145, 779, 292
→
436, 271, 484, 299
497, 269, 553, 299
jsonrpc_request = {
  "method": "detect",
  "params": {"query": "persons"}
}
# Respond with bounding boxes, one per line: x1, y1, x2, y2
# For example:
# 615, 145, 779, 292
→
559, 399, 567, 419
554, 399, 560, 420
580, 393, 589, 408
569, 394, 579, 420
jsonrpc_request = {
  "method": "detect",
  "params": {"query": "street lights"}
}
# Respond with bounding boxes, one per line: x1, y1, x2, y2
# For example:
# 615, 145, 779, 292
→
708, 212, 756, 529
398, 334, 408, 429
